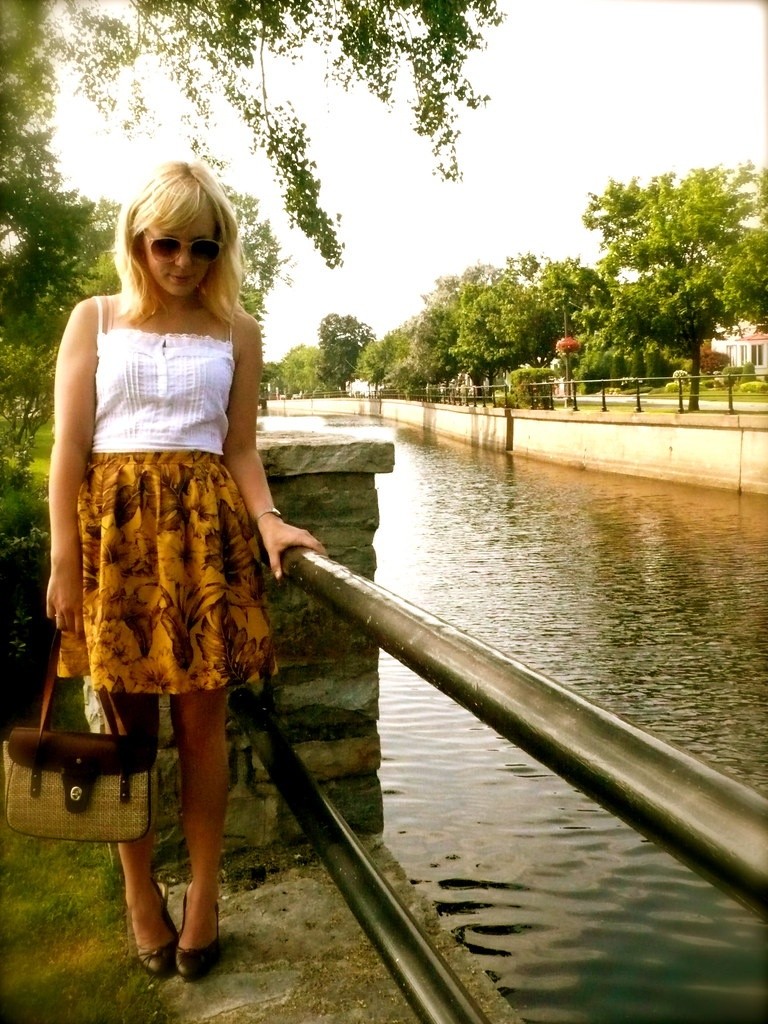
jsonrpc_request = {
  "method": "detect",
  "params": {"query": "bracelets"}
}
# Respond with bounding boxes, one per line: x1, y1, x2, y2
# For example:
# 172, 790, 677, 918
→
254, 507, 282, 523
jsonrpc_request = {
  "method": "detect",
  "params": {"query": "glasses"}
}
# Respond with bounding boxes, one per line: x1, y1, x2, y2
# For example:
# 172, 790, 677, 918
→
143, 229, 223, 264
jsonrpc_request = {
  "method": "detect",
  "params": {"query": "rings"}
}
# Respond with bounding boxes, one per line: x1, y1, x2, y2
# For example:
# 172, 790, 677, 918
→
53, 614, 64, 619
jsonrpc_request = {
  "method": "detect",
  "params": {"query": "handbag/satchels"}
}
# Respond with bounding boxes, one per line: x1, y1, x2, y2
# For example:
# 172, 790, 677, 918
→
3, 629, 158, 842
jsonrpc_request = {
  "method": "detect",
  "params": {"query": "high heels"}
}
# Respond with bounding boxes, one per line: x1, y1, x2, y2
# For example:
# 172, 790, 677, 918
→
174, 880, 221, 982
129, 877, 180, 978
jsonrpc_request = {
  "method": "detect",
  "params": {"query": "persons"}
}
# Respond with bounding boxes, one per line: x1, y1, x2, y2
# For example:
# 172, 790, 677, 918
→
45, 160, 328, 982
